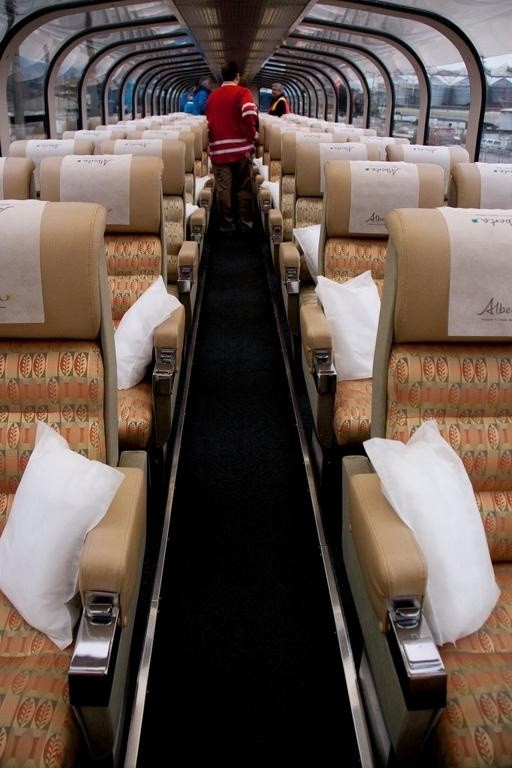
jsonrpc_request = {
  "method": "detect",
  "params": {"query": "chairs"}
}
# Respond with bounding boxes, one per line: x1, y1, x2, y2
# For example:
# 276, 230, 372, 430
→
253, 115, 509, 765
0, 114, 214, 765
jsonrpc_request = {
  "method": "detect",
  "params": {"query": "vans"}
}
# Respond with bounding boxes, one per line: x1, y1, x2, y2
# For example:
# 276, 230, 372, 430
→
480, 137, 504, 150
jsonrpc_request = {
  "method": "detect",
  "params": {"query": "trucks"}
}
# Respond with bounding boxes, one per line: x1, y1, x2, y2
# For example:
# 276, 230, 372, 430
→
430, 126, 455, 146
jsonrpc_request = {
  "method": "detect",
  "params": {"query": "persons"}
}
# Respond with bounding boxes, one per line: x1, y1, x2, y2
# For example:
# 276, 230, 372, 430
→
180, 86, 196, 112
206, 61, 261, 239
192, 76, 214, 115
267, 83, 290, 117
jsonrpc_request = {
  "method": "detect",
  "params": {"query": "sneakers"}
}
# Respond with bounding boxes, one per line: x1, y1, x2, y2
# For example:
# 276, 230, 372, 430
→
219, 216, 253, 230
